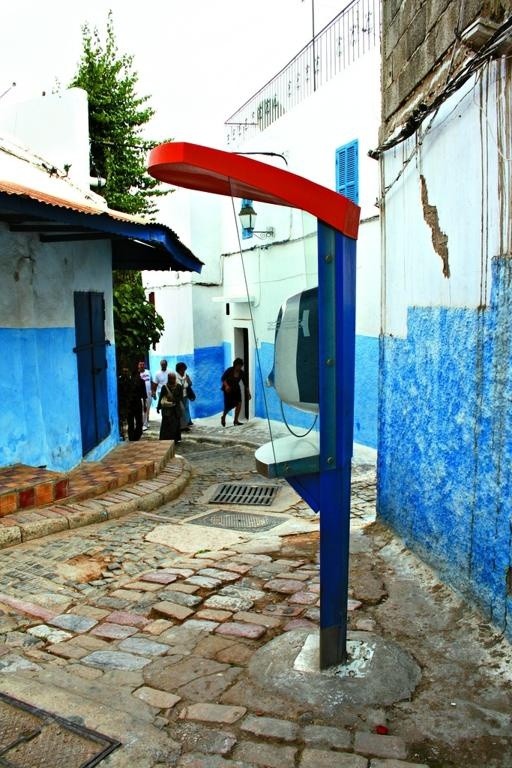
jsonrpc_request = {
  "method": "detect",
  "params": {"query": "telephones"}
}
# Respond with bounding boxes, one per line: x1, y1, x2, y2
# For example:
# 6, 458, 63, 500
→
266, 287, 318, 416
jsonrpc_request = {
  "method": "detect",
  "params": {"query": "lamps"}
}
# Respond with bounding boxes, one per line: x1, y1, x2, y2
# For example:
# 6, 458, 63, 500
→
238, 203, 276, 239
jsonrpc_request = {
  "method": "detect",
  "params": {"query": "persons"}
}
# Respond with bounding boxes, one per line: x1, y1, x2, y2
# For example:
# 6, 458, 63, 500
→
119, 367, 131, 421
126, 379, 148, 441
138, 361, 151, 431
156, 371, 182, 445
175, 362, 193, 432
220, 357, 251, 427
151, 359, 171, 400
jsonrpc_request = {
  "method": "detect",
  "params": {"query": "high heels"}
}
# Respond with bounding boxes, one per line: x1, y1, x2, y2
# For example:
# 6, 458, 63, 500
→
233, 420, 244, 426
221, 416, 225, 427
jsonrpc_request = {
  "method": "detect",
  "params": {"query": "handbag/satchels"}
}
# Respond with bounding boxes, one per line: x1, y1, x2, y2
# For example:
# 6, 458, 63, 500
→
186, 386, 196, 402
160, 394, 177, 409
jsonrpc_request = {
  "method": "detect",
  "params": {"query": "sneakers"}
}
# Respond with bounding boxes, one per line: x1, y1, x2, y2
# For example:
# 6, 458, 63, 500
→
147, 422, 150, 428
142, 425, 148, 431
